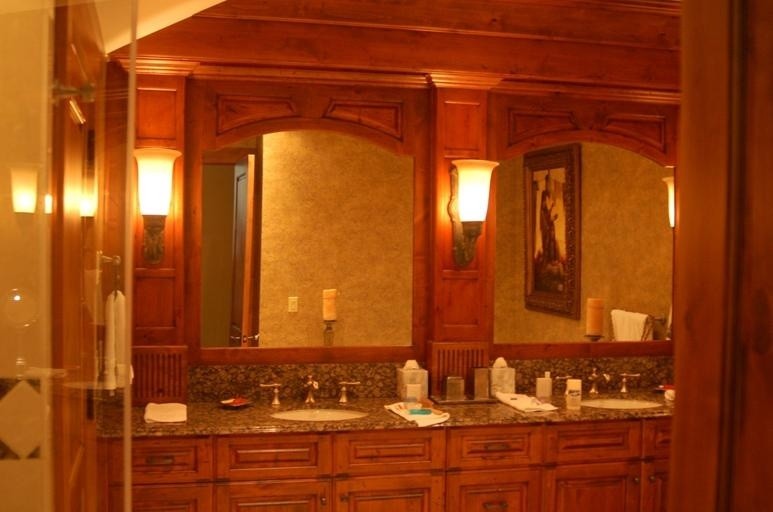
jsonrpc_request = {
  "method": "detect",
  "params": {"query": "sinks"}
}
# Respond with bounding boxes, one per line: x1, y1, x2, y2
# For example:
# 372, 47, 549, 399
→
584, 398, 660, 409
273, 407, 367, 423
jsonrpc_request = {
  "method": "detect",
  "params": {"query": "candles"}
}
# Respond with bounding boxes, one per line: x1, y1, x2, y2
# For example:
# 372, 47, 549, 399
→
320, 288, 339, 320
584, 298, 606, 337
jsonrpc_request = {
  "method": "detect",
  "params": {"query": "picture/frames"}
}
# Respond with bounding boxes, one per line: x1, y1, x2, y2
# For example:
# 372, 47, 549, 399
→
523, 145, 582, 320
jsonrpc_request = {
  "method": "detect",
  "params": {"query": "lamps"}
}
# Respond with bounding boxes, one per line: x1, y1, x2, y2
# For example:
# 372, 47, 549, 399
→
10, 170, 37, 213
661, 176, 676, 228
448, 157, 500, 267
77, 184, 97, 219
131, 148, 182, 265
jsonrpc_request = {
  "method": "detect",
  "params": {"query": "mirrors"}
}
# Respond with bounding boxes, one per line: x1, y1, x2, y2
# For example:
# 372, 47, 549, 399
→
493, 141, 676, 346
199, 128, 415, 348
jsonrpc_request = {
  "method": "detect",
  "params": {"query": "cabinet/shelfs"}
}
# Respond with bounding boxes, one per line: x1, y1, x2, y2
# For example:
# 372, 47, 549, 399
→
543, 418, 672, 511
109, 439, 211, 511
445, 428, 544, 511
215, 430, 445, 512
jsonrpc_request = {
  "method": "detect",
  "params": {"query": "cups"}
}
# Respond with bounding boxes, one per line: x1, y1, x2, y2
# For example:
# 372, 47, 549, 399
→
567, 379, 582, 411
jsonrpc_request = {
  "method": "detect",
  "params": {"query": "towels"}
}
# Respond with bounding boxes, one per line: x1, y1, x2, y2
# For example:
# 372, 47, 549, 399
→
105, 288, 134, 395
144, 401, 188, 424
495, 391, 559, 413
610, 309, 653, 343
383, 399, 450, 430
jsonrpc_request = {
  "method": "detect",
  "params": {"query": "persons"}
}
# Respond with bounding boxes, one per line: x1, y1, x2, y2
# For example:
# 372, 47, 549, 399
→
540, 189, 560, 260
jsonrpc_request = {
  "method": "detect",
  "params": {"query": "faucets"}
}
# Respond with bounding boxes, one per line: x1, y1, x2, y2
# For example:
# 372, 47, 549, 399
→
586, 366, 613, 394
304, 374, 319, 405
